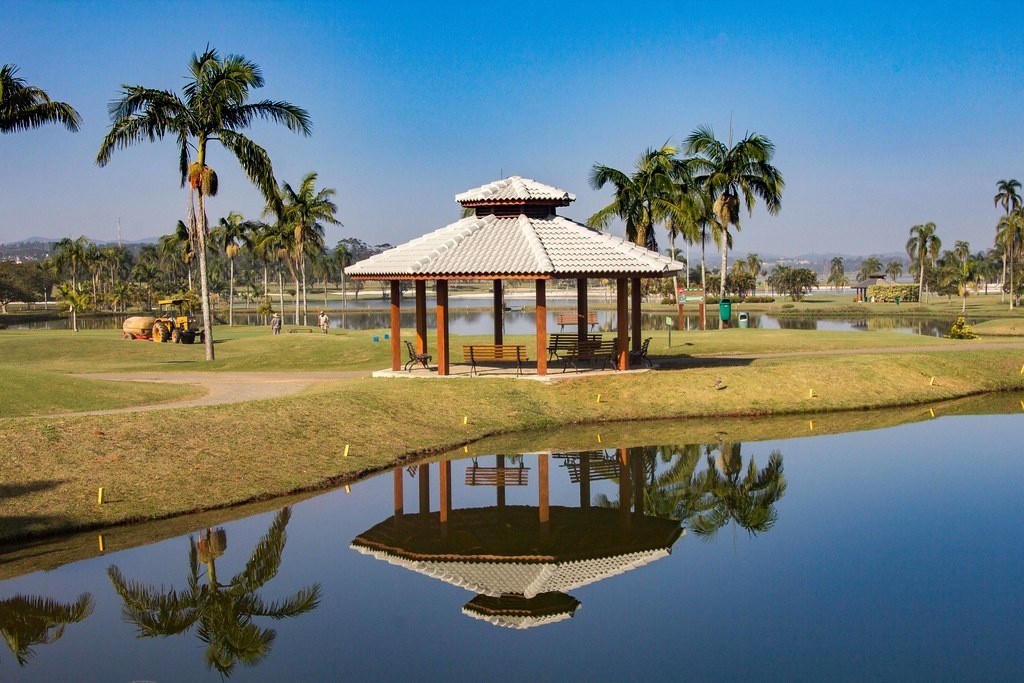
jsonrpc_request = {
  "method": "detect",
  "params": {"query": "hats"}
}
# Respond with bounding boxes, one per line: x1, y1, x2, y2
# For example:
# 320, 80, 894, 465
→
273, 313, 280, 317
320, 311, 324, 314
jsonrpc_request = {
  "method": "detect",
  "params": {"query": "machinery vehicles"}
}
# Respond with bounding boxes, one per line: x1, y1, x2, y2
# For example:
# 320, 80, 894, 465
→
151, 299, 205, 344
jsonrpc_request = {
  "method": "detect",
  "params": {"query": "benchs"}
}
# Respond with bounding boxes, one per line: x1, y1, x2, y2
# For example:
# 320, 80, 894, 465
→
603, 337, 631, 370
552, 450, 620, 484
466, 454, 530, 486
546, 334, 602, 364
407, 465, 418, 478
404, 341, 432, 372
628, 337, 653, 368
557, 312, 599, 333
558, 340, 617, 373
463, 344, 529, 377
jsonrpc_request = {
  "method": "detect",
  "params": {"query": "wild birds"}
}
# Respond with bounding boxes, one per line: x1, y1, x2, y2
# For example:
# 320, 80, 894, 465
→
713, 377, 723, 389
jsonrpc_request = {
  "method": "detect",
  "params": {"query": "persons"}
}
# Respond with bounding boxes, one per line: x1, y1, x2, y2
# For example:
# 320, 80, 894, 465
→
270, 312, 281, 335
316, 310, 329, 334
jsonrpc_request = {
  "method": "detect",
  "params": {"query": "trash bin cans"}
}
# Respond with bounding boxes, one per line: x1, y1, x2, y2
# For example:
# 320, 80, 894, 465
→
719, 299, 731, 321
738, 312, 749, 328
